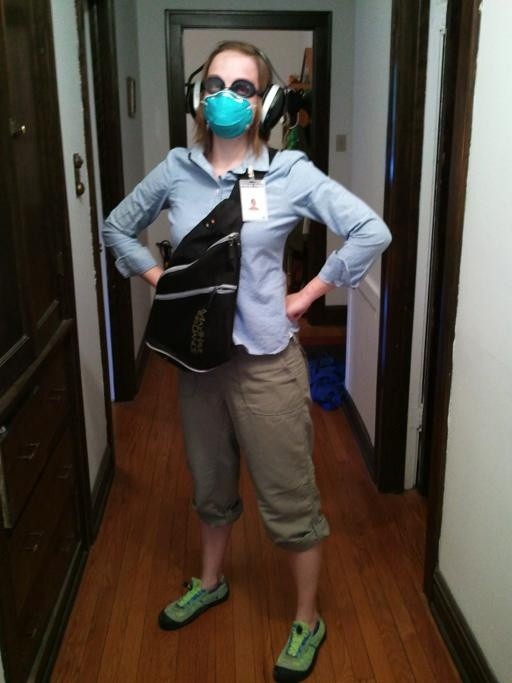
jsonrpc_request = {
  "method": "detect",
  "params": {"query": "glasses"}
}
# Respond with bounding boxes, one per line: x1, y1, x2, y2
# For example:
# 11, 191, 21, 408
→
204, 77, 264, 100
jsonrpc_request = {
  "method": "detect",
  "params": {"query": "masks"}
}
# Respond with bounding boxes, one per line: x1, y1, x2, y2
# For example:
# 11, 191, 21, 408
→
199, 89, 257, 138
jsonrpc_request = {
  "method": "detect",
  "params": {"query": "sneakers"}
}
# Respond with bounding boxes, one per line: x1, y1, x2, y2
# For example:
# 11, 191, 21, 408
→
159, 576, 229, 631
274, 618, 327, 683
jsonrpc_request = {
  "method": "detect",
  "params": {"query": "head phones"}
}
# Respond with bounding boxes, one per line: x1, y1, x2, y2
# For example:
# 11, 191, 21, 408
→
282, 88, 303, 129
182, 59, 285, 139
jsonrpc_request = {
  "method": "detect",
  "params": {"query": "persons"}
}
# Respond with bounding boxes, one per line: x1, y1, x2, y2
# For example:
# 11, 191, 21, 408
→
248, 197, 259, 211
101, 40, 392, 683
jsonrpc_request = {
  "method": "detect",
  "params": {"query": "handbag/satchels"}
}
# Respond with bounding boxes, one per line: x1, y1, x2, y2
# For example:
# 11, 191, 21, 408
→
139, 200, 243, 374
